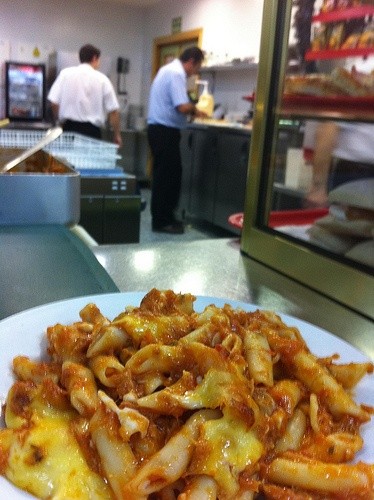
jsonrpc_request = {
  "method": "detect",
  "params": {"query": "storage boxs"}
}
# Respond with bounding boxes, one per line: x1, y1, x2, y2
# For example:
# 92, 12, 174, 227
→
0, 145, 81, 227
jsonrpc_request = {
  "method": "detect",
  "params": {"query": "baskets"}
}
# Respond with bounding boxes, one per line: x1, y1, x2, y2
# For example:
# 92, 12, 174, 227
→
0, 130, 122, 170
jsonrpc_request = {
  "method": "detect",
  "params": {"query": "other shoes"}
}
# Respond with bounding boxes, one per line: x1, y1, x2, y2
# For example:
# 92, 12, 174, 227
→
161, 218, 186, 235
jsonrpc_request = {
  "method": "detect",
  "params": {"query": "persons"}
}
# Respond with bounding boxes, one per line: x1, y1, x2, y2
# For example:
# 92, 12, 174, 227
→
45, 45, 123, 150
147, 47, 204, 235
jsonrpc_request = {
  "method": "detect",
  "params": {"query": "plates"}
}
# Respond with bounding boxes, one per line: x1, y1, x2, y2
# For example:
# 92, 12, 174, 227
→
0, 290, 374, 500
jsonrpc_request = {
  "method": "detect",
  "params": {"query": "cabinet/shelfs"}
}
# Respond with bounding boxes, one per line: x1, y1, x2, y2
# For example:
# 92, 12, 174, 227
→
179, 123, 254, 237
